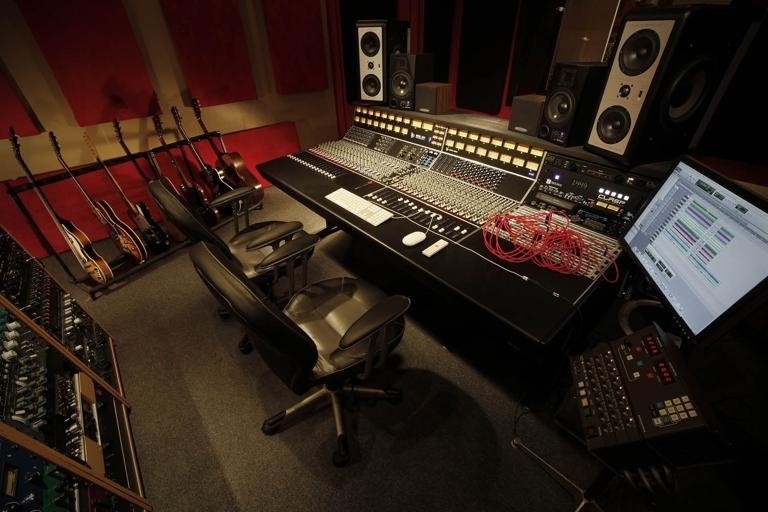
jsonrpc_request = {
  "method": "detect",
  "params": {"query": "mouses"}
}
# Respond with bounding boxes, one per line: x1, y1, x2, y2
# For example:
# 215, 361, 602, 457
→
401, 230, 427, 246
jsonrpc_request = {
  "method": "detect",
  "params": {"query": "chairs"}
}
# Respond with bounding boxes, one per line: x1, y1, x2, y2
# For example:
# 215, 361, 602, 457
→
189, 231, 412, 467
146, 176, 314, 356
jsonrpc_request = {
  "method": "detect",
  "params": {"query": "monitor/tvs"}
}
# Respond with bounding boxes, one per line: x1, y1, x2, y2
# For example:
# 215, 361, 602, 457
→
618, 151, 767, 344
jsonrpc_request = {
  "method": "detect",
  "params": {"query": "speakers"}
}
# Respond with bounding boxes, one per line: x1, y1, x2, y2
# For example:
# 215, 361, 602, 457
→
508, 94, 546, 138
537, 61, 609, 148
415, 82, 450, 114
351, 20, 394, 105
388, 53, 434, 112
582, 4, 744, 171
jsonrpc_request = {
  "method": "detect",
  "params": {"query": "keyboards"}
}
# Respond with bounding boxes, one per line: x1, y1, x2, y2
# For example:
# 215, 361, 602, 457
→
324, 187, 394, 227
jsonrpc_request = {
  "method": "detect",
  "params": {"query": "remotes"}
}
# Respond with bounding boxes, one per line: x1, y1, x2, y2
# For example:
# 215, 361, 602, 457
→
422, 239, 449, 257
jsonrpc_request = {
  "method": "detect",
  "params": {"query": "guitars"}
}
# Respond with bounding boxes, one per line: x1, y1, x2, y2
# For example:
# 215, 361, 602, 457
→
6, 117, 186, 284
151, 98, 264, 225
